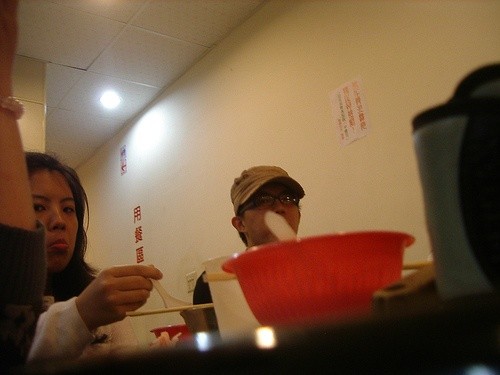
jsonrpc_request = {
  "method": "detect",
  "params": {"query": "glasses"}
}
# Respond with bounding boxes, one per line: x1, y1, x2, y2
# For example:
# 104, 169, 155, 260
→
239, 190, 299, 214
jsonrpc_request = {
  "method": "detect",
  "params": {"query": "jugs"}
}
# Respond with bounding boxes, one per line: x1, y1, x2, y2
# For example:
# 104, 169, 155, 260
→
410, 61, 500, 306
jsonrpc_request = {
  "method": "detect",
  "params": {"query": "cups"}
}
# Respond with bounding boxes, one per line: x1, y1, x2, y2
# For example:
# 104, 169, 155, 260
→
203, 254, 261, 344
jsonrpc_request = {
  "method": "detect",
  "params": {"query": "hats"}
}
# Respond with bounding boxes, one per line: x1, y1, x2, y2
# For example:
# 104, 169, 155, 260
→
231, 165, 305, 216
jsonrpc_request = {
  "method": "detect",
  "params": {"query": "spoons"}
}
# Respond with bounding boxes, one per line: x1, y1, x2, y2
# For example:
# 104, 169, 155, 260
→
150, 278, 193, 308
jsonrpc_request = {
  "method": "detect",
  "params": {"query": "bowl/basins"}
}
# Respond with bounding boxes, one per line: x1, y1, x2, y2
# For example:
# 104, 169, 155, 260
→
222, 230, 415, 332
179, 306, 218, 337
149, 324, 194, 345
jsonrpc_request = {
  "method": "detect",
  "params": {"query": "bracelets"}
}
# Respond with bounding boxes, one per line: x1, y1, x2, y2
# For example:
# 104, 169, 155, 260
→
0, 96, 26, 119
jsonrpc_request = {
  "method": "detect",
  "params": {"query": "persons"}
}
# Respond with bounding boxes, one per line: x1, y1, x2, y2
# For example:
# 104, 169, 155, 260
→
24, 152, 164, 375
0, 0, 48, 375
193, 165, 305, 334
